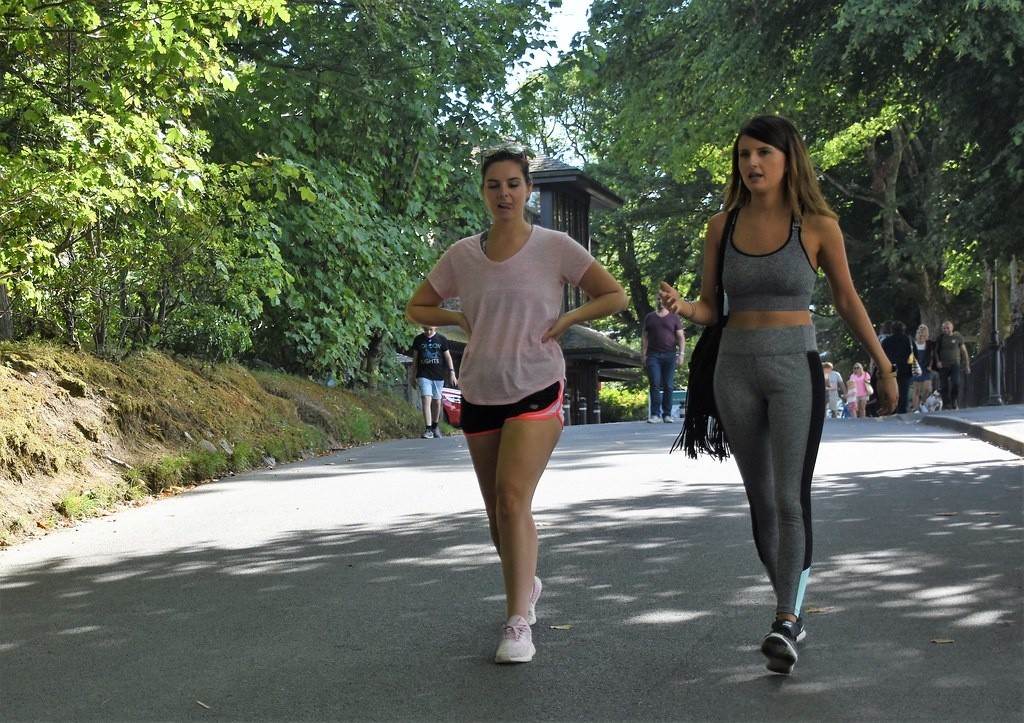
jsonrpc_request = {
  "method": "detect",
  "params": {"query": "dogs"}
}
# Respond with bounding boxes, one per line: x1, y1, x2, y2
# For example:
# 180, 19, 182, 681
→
925, 388, 943, 411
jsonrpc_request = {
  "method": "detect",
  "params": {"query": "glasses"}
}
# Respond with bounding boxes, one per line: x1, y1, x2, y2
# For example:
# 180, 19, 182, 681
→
854, 367, 860, 370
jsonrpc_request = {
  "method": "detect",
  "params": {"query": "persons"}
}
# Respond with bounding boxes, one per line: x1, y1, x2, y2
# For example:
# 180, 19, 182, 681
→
410, 324, 459, 439
640, 293, 686, 424
659, 115, 899, 674
870, 319, 970, 417
406, 150, 629, 663
821, 362, 871, 418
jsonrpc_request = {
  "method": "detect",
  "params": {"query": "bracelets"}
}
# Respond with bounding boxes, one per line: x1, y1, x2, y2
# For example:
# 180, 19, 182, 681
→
685, 300, 696, 321
679, 352, 684, 355
449, 368, 454, 374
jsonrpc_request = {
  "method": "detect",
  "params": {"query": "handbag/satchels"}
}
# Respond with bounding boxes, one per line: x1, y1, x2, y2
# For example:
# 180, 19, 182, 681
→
665, 327, 733, 458
864, 372, 874, 395
909, 336, 922, 377
932, 334, 943, 371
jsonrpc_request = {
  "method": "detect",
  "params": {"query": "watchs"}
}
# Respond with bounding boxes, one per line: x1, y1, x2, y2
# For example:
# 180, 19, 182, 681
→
876, 364, 899, 378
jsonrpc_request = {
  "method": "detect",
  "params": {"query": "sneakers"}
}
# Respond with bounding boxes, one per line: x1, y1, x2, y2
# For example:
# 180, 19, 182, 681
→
764, 617, 806, 642
521, 575, 541, 625
421, 429, 434, 439
432, 426, 442, 439
761, 620, 798, 676
495, 616, 536, 663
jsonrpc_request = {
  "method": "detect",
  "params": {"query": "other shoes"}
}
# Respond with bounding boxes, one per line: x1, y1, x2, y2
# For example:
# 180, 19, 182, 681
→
919, 403, 928, 413
648, 415, 661, 423
913, 409, 920, 414
663, 416, 673, 423
